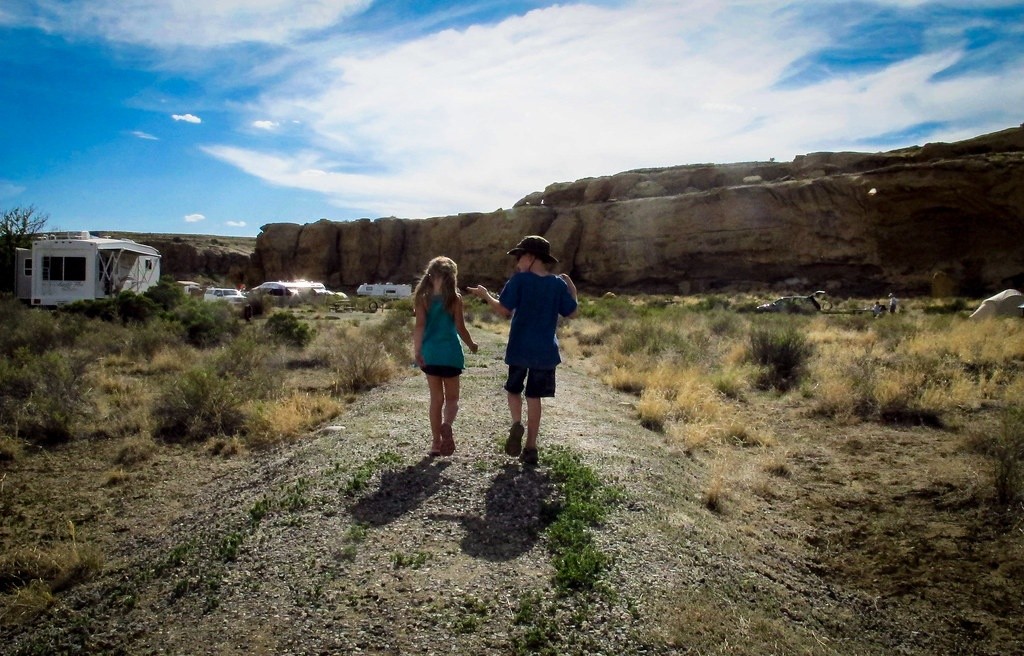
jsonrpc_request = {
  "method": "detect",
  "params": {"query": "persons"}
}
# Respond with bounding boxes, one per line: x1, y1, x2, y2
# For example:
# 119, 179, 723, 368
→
888, 293, 897, 313
413, 255, 479, 455
467, 234, 578, 463
873, 301, 881, 315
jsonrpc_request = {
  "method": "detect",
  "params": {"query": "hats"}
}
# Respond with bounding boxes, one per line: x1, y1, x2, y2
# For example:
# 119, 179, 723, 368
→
507, 234, 559, 265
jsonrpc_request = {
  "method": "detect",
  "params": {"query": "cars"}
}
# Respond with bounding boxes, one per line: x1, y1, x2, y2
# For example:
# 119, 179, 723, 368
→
756, 295, 817, 318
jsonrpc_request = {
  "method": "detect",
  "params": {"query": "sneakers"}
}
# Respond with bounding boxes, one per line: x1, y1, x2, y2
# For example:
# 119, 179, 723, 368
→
440, 421, 455, 456
504, 422, 525, 457
431, 439, 441, 456
519, 446, 539, 464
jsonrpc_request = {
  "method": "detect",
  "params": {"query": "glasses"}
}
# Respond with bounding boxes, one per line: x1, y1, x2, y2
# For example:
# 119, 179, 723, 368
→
516, 250, 528, 261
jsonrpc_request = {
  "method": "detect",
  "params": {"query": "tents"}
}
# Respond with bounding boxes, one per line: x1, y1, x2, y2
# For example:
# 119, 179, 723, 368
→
967, 288, 1023, 323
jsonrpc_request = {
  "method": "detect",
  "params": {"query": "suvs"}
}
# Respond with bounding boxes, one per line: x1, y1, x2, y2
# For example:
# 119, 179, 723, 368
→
203, 287, 247, 313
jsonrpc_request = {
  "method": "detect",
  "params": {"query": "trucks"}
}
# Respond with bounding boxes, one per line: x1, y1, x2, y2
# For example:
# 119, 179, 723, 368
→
249, 280, 351, 318
14, 231, 163, 311
357, 282, 414, 314
